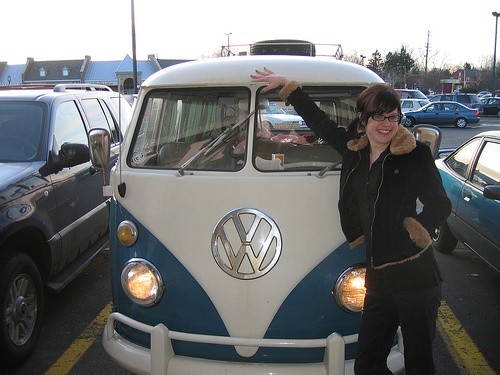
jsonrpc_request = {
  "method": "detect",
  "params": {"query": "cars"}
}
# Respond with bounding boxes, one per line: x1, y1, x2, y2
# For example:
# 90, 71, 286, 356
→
400, 99, 430, 113
477, 90, 499, 99
435, 129, 500, 276
480, 96, 500, 118
259, 102, 309, 131
400, 100, 480, 128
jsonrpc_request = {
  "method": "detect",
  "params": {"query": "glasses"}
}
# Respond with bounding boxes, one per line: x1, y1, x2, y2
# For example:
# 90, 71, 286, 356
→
370, 114, 402, 122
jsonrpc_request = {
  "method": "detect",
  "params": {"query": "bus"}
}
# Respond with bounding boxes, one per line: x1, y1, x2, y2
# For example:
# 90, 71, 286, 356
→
88, 40, 443, 374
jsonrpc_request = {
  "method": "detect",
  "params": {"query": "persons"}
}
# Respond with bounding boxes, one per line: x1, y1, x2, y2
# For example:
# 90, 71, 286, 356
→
250, 64, 453, 375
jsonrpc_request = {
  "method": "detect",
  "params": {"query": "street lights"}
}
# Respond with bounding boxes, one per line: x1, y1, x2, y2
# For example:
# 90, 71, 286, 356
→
491, 11, 500, 97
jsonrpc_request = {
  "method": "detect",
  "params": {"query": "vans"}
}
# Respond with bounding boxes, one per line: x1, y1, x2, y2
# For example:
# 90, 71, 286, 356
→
429, 93, 483, 113
393, 88, 427, 100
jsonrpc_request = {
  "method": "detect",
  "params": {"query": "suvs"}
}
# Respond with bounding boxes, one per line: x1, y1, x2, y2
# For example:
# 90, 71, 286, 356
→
0, 83, 135, 367
